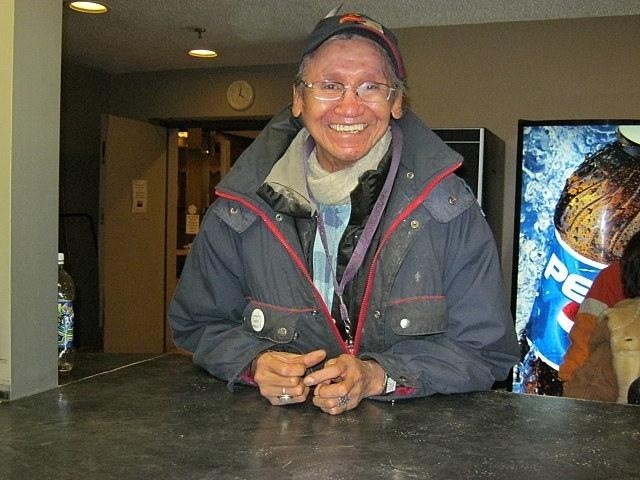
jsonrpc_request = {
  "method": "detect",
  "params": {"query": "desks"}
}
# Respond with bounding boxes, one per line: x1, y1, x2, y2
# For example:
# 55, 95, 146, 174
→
0, 352, 640, 480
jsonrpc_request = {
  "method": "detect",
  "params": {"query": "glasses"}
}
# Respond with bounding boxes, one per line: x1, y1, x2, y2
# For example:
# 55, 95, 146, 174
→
298, 79, 396, 104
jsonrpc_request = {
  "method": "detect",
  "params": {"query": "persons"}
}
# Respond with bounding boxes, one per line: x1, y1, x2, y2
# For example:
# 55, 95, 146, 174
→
557, 260, 627, 399
161, 13, 523, 415
567, 227, 640, 409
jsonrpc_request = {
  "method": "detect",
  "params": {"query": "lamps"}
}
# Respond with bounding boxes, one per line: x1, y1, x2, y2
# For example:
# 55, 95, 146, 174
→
64, 0, 218, 60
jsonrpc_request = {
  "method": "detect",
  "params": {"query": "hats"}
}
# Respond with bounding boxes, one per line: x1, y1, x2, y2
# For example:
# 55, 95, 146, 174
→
299, 12, 407, 79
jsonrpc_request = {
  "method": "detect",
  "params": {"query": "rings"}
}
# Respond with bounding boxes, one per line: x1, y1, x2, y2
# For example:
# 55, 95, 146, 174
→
281, 387, 287, 396
338, 393, 349, 407
278, 394, 294, 400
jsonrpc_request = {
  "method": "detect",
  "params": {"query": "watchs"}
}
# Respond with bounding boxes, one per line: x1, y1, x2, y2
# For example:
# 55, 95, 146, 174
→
375, 371, 398, 397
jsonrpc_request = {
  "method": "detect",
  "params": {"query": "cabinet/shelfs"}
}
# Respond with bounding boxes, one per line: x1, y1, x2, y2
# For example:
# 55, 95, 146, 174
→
426, 127, 504, 270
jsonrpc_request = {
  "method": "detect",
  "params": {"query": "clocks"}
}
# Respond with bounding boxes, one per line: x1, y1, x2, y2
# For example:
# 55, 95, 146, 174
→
226, 79, 255, 110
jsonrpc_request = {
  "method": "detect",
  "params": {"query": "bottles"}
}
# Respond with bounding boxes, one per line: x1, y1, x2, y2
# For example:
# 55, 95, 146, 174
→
515, 124, 640, 397
58, 253, 77, 377
